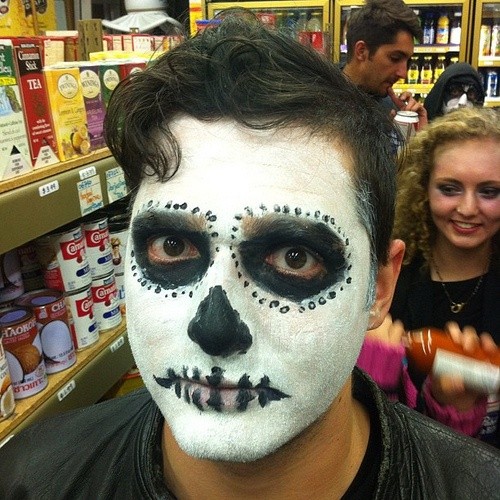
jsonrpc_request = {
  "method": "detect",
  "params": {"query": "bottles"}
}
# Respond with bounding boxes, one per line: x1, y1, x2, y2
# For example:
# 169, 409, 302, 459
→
273, 11, 463, 85
399, 330, 500, 391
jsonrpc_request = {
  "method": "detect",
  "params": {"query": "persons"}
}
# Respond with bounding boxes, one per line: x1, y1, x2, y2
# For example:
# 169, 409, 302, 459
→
0, 0, 500, 500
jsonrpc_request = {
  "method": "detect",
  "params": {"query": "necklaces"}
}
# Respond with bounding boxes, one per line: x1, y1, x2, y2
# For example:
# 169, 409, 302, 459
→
427, 245, 494, 314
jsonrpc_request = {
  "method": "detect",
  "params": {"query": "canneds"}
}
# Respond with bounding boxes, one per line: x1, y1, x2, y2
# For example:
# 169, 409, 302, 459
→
478, 71, 485, 92
485, 72, 498, 97
0, 210, 131, 422
479, 25, 500, 57
392, 110, 419, 141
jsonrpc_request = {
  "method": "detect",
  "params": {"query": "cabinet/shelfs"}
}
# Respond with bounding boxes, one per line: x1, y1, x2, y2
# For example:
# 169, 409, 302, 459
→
1, 0, 499, 447
